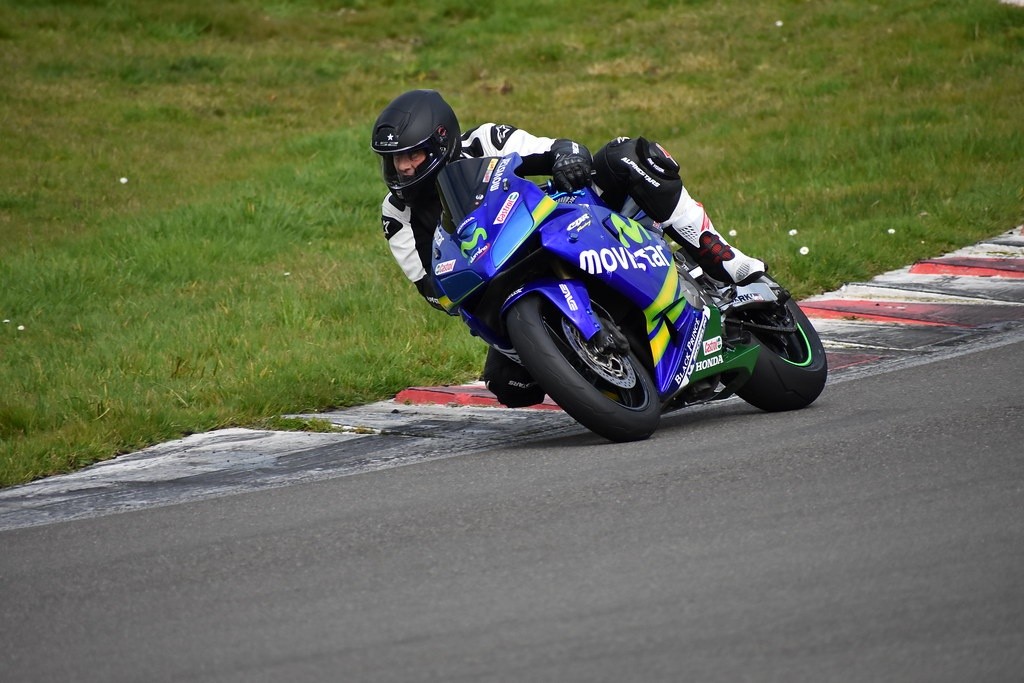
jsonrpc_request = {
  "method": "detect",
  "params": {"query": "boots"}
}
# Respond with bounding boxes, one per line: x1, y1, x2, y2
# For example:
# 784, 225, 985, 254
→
660, 201, 769, 287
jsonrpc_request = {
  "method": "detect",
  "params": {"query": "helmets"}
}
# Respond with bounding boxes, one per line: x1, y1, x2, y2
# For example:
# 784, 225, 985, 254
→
371, 89, 463, 203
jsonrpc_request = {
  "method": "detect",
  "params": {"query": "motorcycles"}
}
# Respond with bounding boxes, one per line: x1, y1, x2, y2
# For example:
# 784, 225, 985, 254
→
428, 151, 829, 443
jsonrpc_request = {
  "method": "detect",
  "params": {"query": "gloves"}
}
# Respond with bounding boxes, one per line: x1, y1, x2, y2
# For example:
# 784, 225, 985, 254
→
550, 138, 594, 195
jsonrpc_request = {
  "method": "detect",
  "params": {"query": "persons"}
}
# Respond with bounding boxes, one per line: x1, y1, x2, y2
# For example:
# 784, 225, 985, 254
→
372, 88, 768, 409
